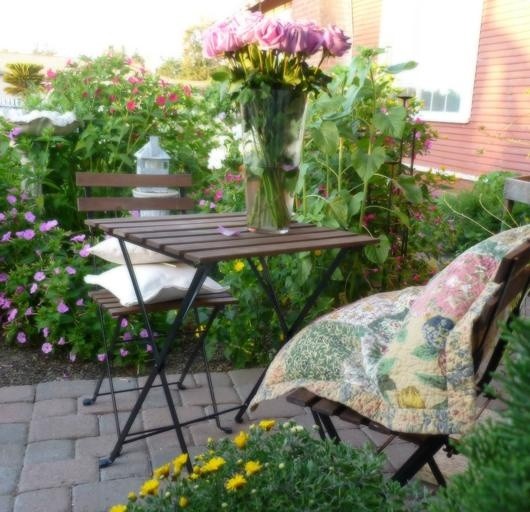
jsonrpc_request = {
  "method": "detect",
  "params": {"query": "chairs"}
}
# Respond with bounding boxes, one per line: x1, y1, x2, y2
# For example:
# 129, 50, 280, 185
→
71, 168, 236, 472
288, 230, 529, 490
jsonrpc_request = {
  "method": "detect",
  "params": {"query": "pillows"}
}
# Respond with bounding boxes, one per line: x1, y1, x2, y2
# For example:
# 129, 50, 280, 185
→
247, 220, 528, 439
83, 262, 232, 308
86, 234, 189, 265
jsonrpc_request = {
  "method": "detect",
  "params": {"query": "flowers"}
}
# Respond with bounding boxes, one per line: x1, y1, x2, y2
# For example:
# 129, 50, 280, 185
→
202, 10, 353, 232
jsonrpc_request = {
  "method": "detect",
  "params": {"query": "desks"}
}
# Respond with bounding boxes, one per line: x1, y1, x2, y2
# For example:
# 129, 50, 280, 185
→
84, 211, 383, 477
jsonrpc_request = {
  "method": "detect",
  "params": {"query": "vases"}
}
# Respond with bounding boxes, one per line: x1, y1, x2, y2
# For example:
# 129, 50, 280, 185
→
239, 86, 310, 232
